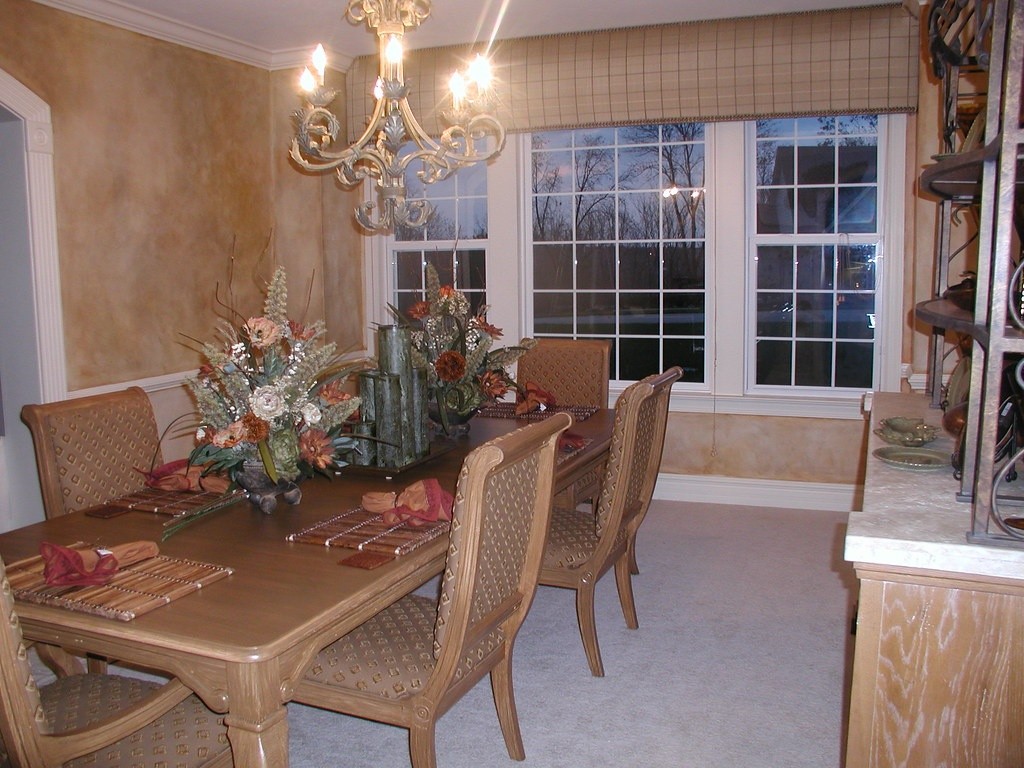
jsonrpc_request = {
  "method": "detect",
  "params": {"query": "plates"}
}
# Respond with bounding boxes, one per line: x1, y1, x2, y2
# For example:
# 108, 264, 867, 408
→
872, 429, 937, 445
872, 446, 952, 471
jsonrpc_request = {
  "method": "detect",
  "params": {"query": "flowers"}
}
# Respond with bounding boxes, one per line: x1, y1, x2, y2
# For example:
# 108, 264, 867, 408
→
366, 226, 557, 425
150, 225, 401, 492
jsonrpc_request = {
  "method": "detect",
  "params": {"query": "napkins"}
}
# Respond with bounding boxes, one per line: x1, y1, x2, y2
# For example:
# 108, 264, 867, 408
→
361, 477, 454, 528
133, 459, 231, 494
559, 433, 585, 452
40, 540, 160, 588
515, 382, 557, 416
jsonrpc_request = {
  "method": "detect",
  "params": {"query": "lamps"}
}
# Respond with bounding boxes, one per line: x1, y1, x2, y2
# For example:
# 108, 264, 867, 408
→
287, 0, 508, 232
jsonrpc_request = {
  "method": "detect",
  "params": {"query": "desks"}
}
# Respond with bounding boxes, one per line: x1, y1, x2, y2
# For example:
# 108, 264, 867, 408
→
0, 402, 640, 768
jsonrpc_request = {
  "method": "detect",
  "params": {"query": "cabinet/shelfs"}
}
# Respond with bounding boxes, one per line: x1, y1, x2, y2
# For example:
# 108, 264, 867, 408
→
842, 389, 1024, 768
912, 0, 1024, 509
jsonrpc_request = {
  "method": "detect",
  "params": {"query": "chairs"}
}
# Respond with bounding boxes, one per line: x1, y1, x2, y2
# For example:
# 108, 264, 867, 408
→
21, 386, 165, 674
516, 338, 613, 514
539, 366, 684, 677
0, 556, 234, 768
290, 410, 576, 768
